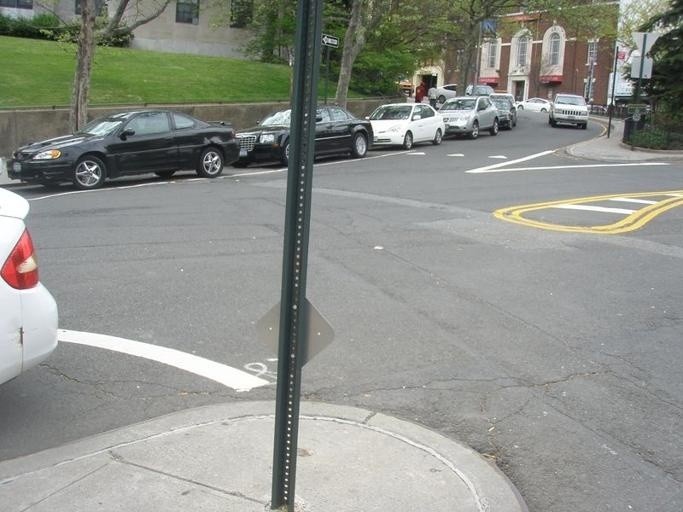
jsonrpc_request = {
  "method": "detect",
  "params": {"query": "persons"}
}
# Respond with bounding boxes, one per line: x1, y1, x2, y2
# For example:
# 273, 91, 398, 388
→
415, 81, 426, 103
427, 83, 438, 111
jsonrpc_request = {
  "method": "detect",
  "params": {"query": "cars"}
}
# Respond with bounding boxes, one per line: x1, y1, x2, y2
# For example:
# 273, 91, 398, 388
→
514, 96, 551, 113
488, 93, 516, 130
436, 83, 466, 103
234, 105, 373, 167
0, 185, 58, 386
438, 96, 500, 139
365, 102, 445, 150
7, 109, 241, 190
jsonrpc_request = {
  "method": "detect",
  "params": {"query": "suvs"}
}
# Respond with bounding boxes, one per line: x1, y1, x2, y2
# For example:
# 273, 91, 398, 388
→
465, 83, 494, 97
548, 93, 589, 129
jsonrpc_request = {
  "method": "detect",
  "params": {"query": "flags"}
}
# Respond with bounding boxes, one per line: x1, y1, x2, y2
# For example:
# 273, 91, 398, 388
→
480, 5, 497, 39
617, 46, 627, 63
